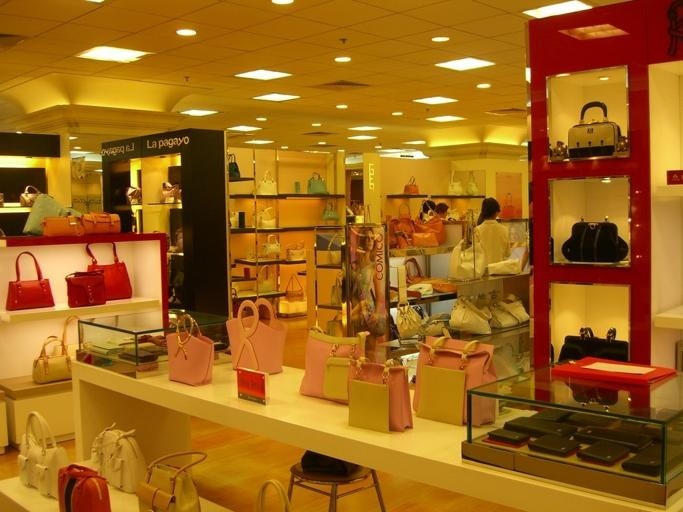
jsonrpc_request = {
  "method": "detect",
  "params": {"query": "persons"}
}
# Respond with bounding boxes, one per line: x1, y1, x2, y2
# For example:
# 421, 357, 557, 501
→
435, 201, 450, 219
422, 200, 437, 219
350, 227, 383, 363
470, 198, 512, 269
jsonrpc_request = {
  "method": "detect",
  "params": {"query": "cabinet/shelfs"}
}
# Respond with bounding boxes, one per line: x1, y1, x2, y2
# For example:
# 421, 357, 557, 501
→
228, 178, 346, 320
528, 0, 683, 402
389, 247, 458, 389
0, 232, 171, 457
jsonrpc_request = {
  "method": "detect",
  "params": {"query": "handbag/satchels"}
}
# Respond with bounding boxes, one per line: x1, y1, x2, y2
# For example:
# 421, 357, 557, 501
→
255, 276, 274, 293
320, 208, 339, 220
388, 282, 530, 337
286, 288, 305, 303
123, 185, 141, 206
166, 314, 215, 386
385, 169, 529, 281
252, 207, 278, 228
557, 327, 629, 363
308, 175, 327, 194
413, 335, 498, 428
160, 182, 180, 205
568, 101, 621, 159
226, 298, 289, 375
33, 315, 83, 384
228, 161, 241, 181
287, 248, 306, 259
346, 360, 414, 433
259, 243, 280, 258
315, 233, 343, 335
300, 326, 370, 406
258, 179, 277, 195
6, 261, 132, 311
18, 411, 209, 512
561, 216, 629, 262
0, 184, 122, 236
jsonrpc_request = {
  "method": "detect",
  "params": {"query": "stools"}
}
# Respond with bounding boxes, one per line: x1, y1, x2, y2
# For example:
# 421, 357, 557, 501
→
287, 459, 385, 512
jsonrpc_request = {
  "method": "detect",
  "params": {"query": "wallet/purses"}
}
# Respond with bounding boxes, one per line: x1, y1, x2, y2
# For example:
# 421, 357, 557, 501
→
488, 410, 682, 478
86, 334, 166, 363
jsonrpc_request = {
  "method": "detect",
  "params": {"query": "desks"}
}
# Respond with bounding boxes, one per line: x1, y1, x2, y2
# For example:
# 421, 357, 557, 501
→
1, 458, 230, 512
